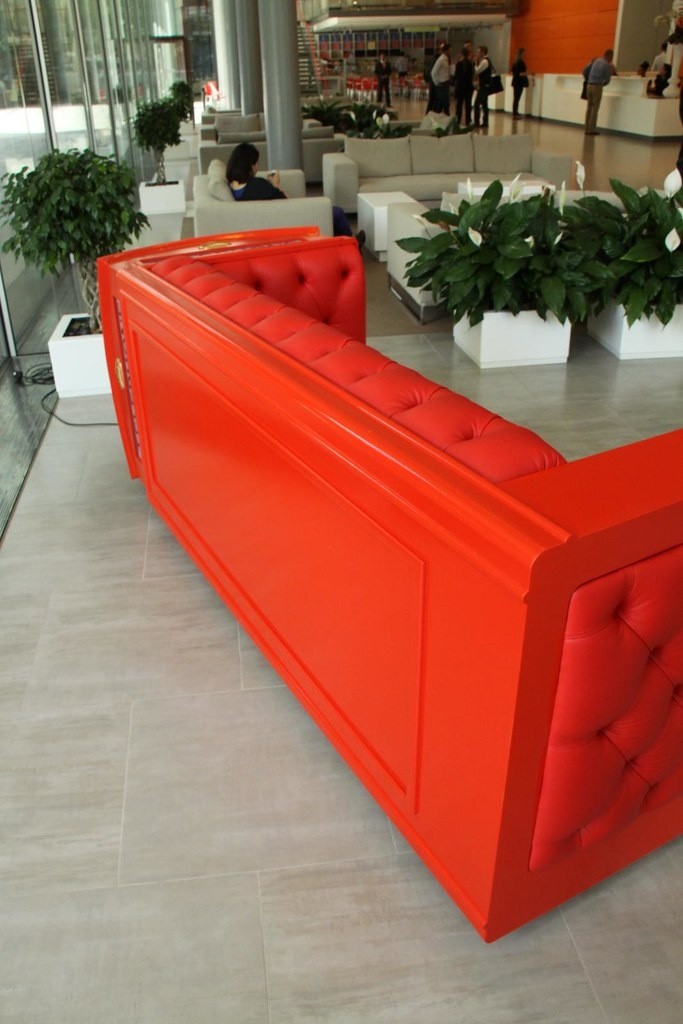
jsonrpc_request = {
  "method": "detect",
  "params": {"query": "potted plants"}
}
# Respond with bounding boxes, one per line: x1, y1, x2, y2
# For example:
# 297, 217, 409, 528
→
169, 82, 194, 135
0, 150, 150, 400
135, 99, 186, 215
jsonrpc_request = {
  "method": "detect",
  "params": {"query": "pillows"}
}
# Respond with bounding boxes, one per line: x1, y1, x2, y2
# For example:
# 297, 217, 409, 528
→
410, 133, 475, 176
260, 112, 265, 130
219, 132, 260, 144
215, 115, 259, 135
341, 137, 416, 180
207, 157, 235, 200
471, 132, 533, 177
208, 107, 217, 114
302, 126, 334, 140
421, 112, 450, 129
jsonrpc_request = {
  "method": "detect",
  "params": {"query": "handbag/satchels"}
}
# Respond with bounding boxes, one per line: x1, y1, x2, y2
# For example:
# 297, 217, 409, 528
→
580, 81, 587, 100
488, 75, 504, 95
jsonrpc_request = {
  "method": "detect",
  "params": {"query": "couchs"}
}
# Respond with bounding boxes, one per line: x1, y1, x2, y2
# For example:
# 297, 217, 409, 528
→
95, 226, 683, 944
302, 119, 468, 182
385, 188, 682, 319
193, 170, 333, 240
201, 109, 241, 124
201, 113, 322, 144
320, 134, 572, 214
198, 126, 339, 183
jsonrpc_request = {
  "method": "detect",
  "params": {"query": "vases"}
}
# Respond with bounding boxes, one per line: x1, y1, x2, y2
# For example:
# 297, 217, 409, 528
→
582, 294, 683, 359
450, 309, 571, 367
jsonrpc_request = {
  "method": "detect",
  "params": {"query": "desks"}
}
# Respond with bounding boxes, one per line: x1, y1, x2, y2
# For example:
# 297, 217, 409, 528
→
472, 72, 682, 141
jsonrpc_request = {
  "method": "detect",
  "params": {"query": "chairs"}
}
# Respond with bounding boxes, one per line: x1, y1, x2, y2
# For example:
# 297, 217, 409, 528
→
345, 74, 427, 103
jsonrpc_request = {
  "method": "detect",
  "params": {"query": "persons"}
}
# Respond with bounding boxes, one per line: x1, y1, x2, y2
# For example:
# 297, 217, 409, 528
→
637, 60, 650, 78
458, 43, 478, 124
423, 40, 446, 115
582, 48, 614, 135
647, 64, 673, 96
432, 43, 452, 116
454, 48, 475, 126
651, 42, 668, 71
374, 53, 394, 108
395, 51, 412, 100
670, 15, 683, 183
226, 142, 367, 251
472, 45, 494, 127
510, 47, 529, 119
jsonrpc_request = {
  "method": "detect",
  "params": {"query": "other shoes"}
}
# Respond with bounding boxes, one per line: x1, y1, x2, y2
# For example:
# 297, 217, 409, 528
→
585, 130, 599, 135
355, 229, 365, 254
513, 115, 521, 120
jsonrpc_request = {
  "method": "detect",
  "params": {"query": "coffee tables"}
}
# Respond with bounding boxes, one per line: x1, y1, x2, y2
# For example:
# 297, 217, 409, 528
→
457, 179, 555, 198
358, 191, 430, 262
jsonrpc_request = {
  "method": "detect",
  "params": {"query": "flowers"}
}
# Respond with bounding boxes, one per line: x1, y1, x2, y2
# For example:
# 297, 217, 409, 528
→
574, 162, 683, 333
395, 164, 586, 328
302, 90, 413, 138
